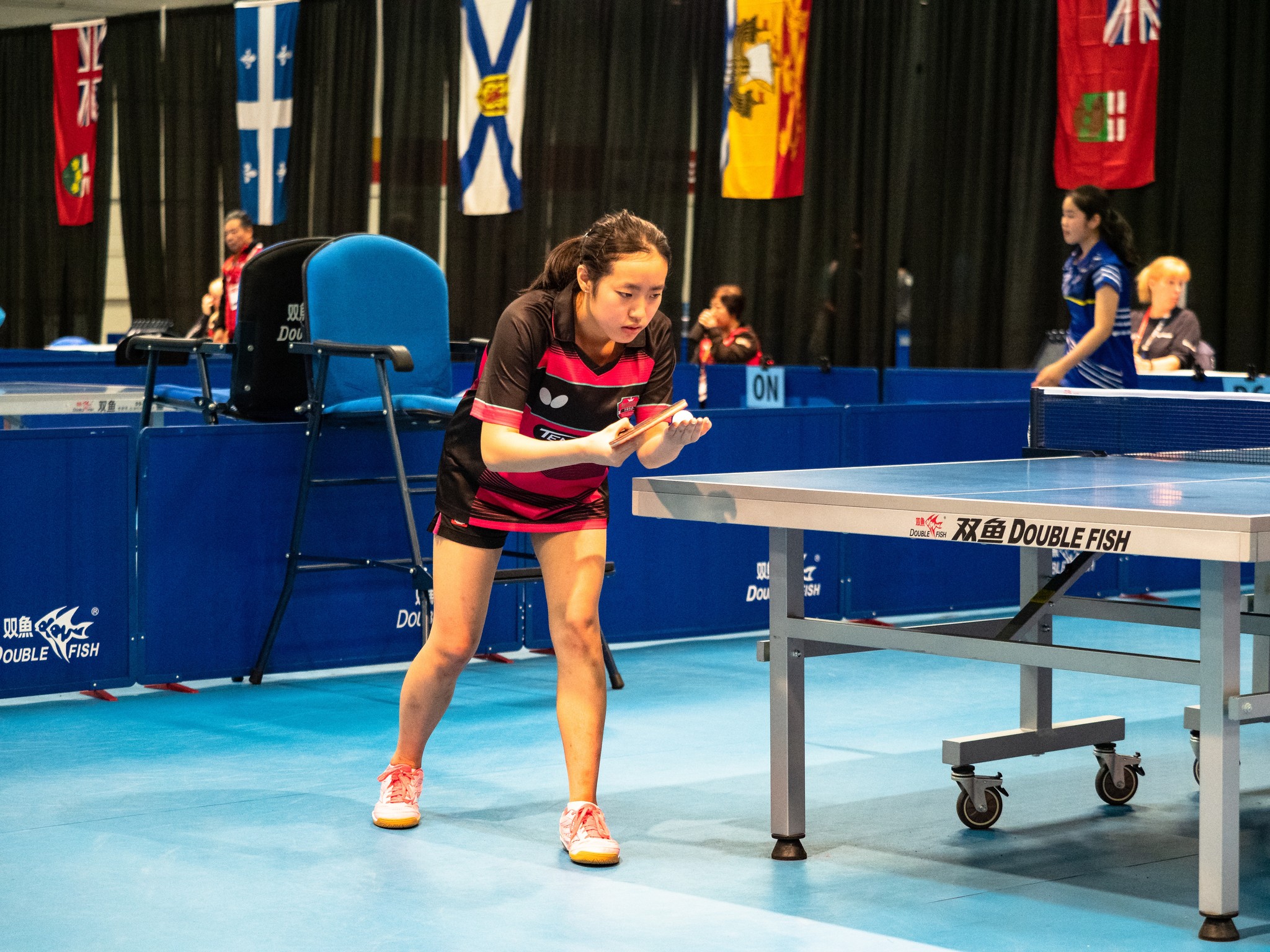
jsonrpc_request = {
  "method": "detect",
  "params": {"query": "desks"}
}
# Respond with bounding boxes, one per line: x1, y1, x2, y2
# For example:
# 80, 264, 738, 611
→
0, 381, 181, 429
635, 449, 1270, 939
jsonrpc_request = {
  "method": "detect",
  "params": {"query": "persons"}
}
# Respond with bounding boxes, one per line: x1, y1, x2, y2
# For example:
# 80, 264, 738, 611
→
687, 284, 763, 366
1128, 256, 1201, 371
193, 211, 266, 344
372, 210, 712, 865
1031, 186, 1140, 388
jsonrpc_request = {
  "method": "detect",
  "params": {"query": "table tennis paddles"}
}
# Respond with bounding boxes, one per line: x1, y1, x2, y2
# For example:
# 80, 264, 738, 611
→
609, 399, 688, 449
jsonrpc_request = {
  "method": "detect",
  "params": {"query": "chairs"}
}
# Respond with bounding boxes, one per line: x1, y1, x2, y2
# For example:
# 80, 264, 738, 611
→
132, 237, 328, 433
250, 234, 631, 690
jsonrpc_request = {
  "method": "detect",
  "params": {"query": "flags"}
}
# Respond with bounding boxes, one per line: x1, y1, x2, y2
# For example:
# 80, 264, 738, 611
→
458, 0, 532, 216
52, 20, 108, 226
719, 0, 811, 199
1054, 0, 1159, 192
233, 0, 298, 226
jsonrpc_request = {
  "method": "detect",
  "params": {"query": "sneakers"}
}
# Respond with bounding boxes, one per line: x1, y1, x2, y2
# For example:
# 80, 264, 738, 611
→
371, 764, 422, 827
559, 801, 620, 867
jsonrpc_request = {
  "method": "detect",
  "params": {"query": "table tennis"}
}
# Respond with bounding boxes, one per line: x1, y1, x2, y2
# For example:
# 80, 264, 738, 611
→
672, 410, 694, 425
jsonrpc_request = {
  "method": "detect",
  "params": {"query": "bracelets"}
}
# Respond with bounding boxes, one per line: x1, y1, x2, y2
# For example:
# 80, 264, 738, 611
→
1149, 360, 1153, 370
